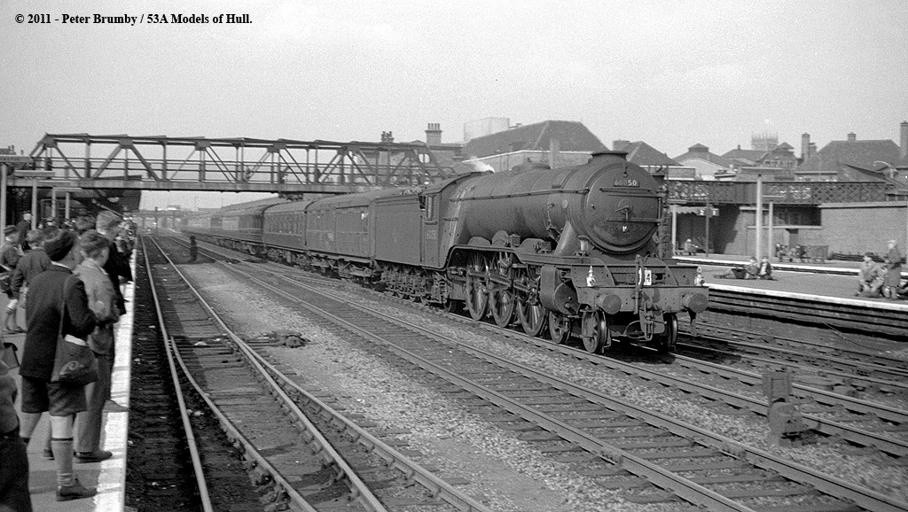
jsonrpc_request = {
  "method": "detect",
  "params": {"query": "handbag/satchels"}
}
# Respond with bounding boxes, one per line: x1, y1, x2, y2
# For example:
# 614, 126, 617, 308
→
50, 337, 100, 384
0, 271, 14, 293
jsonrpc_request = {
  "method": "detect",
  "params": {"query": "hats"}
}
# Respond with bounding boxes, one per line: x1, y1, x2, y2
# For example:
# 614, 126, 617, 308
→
24, 230, 43, 243
3, 225, 16, 234
44, 211, 122, 257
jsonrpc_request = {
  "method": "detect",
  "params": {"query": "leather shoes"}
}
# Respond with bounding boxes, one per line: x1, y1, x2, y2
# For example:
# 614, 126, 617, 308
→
74, 450, 112, 463
55, 478, 98, 501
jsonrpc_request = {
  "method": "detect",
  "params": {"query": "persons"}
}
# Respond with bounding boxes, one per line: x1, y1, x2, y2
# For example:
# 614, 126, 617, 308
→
776, 241, 806, 262
884, 240, 903, 301
1, 209, 137, 512
858, 252, 884, 297
685, 238, 699, 255
716, 256, 774, 280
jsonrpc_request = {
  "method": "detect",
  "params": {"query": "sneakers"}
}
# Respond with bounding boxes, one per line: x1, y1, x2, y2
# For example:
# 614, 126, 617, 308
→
2, 326, 14, 334
15, 326, 27, 334
103, 401, 128, 413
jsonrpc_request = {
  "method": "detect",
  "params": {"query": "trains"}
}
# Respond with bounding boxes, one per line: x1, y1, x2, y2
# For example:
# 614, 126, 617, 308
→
181, 149, 706, 354
